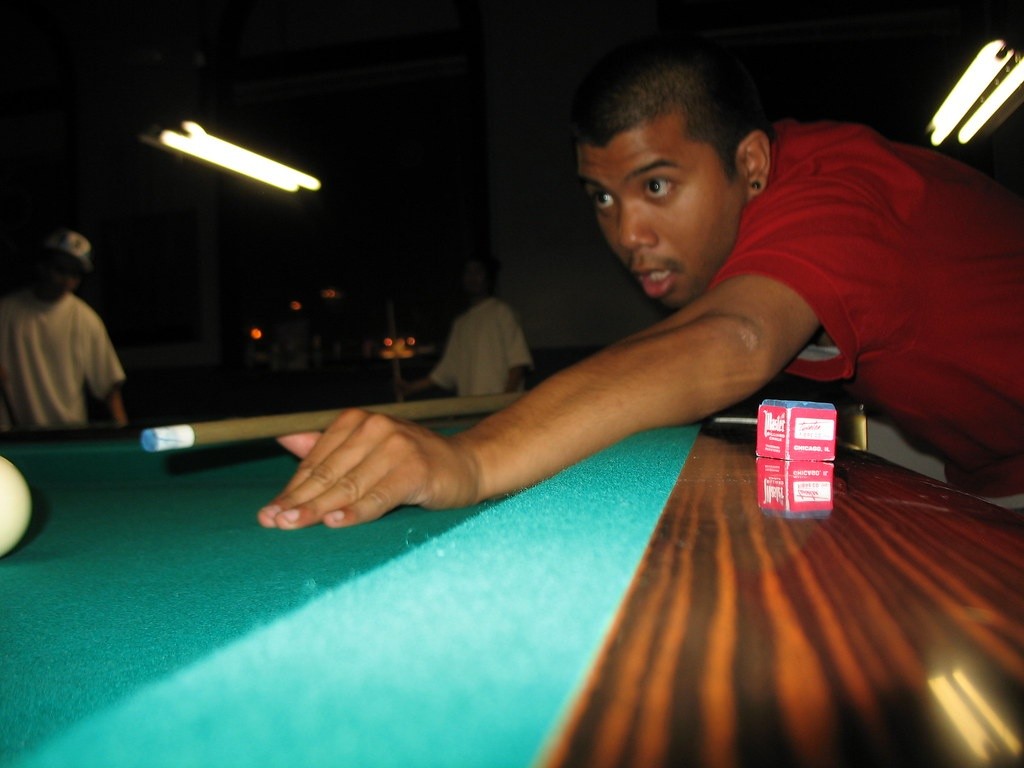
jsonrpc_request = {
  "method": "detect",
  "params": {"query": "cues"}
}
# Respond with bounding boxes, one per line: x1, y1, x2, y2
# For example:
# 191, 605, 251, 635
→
140, 394, 530, 454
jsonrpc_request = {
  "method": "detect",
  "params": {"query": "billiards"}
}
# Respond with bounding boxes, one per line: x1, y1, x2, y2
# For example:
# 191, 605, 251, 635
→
1, 458, 34, 560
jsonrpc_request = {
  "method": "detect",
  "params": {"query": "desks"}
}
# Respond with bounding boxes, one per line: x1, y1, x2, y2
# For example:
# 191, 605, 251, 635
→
0, 421, 1024, 767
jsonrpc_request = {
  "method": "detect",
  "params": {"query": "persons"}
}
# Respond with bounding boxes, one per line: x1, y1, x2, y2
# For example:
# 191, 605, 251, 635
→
389, 265, 535, 401
0, 231, 131, 432
254, 32, 1024, 533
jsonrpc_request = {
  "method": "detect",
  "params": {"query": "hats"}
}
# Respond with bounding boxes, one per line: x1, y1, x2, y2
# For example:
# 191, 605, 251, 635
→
46, 229, 95, 277
